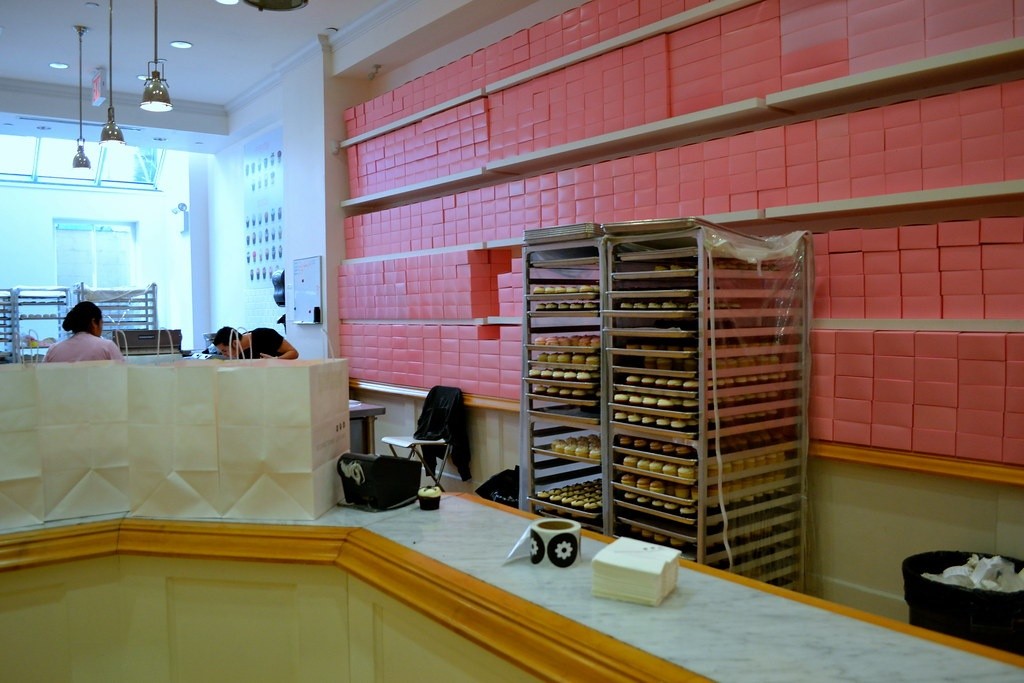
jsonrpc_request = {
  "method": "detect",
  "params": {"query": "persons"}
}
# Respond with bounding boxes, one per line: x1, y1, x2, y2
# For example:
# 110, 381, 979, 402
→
41, 301, 122, 362
213, 327, 299, 359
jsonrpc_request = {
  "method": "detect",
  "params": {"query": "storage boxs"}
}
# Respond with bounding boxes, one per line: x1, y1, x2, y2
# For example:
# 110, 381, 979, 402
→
337, 0, 1024, 467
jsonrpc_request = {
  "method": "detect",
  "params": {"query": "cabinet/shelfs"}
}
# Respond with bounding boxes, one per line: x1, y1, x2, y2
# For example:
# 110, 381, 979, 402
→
339, 0, 1024, 485
520, 218, 816, 596
0, 283, 156, 364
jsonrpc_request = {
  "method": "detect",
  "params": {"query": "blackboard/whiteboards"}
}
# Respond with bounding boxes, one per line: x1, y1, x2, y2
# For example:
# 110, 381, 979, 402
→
291, 255, 324, 325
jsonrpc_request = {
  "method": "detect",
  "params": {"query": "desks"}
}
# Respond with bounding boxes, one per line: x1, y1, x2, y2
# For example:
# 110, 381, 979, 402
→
349, 399, 385, 456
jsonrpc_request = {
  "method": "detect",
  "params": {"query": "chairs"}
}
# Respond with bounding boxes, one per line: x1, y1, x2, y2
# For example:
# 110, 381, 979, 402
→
381, 386, 472, 492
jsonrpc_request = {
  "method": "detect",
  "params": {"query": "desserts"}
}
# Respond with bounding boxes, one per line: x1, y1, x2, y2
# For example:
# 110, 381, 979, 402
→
418, 486, 442, 510
20, 314, 56, 318
528, 265, 799, 586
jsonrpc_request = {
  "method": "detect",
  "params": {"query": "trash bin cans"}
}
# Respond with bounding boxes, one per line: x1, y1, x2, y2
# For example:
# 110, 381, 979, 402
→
902, 551, 1024, 655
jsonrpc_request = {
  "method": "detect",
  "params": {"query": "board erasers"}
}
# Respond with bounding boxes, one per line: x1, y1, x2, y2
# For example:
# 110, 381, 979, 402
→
314, 305, 321, 324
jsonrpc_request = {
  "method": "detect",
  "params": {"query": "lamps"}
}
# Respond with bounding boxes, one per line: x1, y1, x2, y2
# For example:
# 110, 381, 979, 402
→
99, 1, 126, 149
72, 25, 91, 169
140, 0, 173, 112
171, 40, 192, 48
